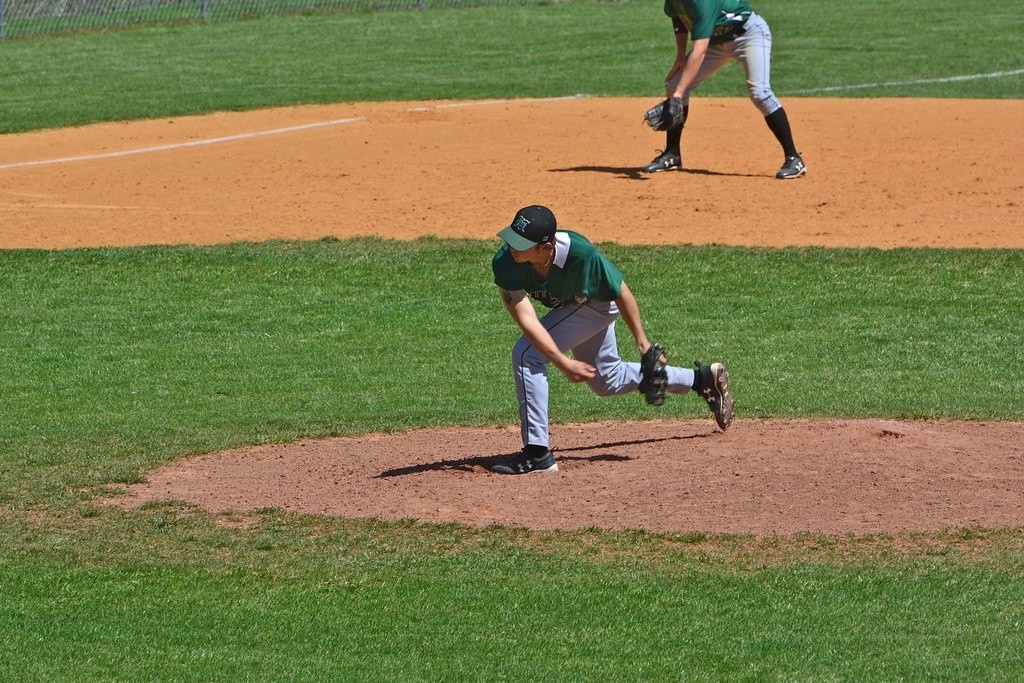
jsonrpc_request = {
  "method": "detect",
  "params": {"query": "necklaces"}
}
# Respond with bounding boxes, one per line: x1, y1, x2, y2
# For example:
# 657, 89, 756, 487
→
544, 258, 550, 267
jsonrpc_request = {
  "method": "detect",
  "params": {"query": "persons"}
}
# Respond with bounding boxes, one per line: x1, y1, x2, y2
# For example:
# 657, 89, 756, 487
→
644, 0, 806, 178
491, 204, 735, 476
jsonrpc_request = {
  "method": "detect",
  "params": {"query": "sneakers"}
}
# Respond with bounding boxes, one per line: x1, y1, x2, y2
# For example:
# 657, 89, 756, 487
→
642, 150, 683, 172
490, 446, 559, 475
776, 155, 808, 178
694, 360, 736, 430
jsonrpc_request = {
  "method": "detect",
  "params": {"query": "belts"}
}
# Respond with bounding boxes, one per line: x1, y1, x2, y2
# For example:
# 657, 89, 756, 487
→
708, 27, 746, 46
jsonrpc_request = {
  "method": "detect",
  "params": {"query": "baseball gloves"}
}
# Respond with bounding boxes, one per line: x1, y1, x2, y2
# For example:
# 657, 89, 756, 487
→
646, 97, 684, 132
637, 340, 669, 406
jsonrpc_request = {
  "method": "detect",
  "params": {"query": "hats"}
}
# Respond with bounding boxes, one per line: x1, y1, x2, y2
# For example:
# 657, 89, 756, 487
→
496, 204, 558, 251
